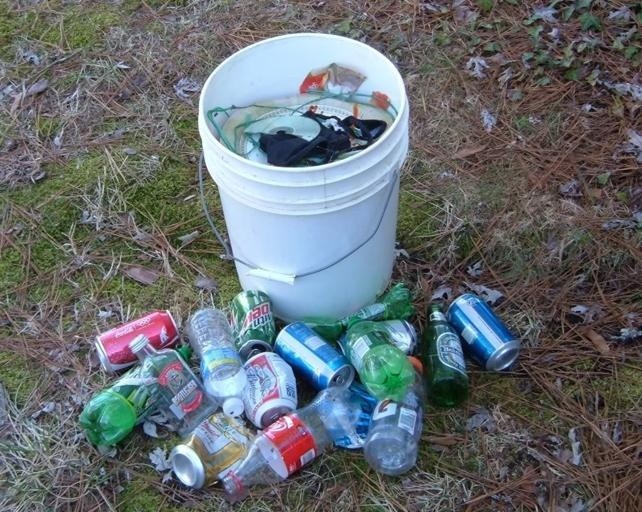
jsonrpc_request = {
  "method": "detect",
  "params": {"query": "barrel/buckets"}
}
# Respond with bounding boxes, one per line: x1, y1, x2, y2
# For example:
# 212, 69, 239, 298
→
198, 32, 410, 321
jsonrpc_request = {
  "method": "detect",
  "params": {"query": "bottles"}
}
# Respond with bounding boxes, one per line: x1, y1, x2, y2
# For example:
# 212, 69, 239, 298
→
347, 317, 417, 398
80, 344, 193, 444
326, 383, 423, 449
188, 309, 249, 416
427, 304, 469, 407
365, 356, 427, 478
223, 386, 362, 495
128, 334, 219, 439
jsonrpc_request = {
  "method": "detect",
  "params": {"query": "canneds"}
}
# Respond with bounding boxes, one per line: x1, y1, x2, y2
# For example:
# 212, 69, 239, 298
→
311, 382, 379, 450
94, 309, 181, 374
445, 294, 520, 374
170, 411, 255, 492
230, 289, 275, 366
273, 319, 355, 393
239, 351, 298, 430
